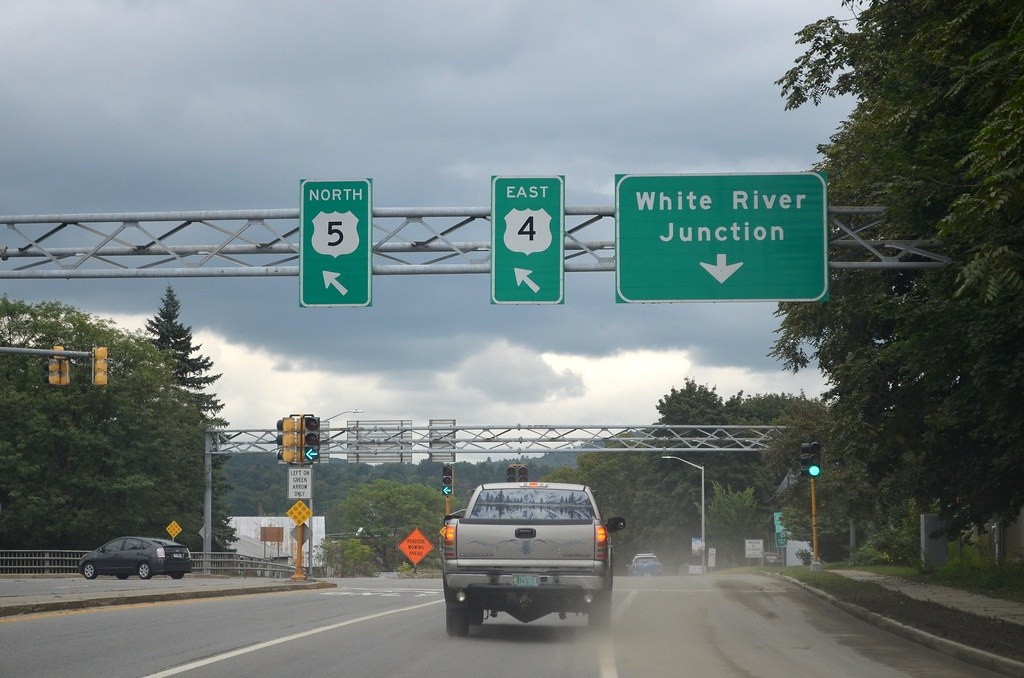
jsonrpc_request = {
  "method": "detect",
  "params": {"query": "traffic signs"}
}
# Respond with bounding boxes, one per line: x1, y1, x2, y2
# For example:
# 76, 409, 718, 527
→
614, 173, 830, 303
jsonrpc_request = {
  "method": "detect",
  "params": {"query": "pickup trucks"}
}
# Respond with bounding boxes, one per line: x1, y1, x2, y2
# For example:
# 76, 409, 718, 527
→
441, 481, 626, 637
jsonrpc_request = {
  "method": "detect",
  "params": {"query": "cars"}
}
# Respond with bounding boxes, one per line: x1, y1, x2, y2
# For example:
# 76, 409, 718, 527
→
77, 536, 194, 579
626, 553, 664, 576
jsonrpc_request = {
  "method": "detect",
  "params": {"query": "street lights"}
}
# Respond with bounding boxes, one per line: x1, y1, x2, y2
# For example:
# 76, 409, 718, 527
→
662, 455, 705, 575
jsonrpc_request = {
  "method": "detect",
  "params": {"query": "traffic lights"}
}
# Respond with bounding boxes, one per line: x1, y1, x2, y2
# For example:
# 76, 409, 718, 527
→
442, 467, 452, 496
44, 359, 59, 384
800, 442, 821, 478
272, 418, 295, 461
302, 416, 321, 463
